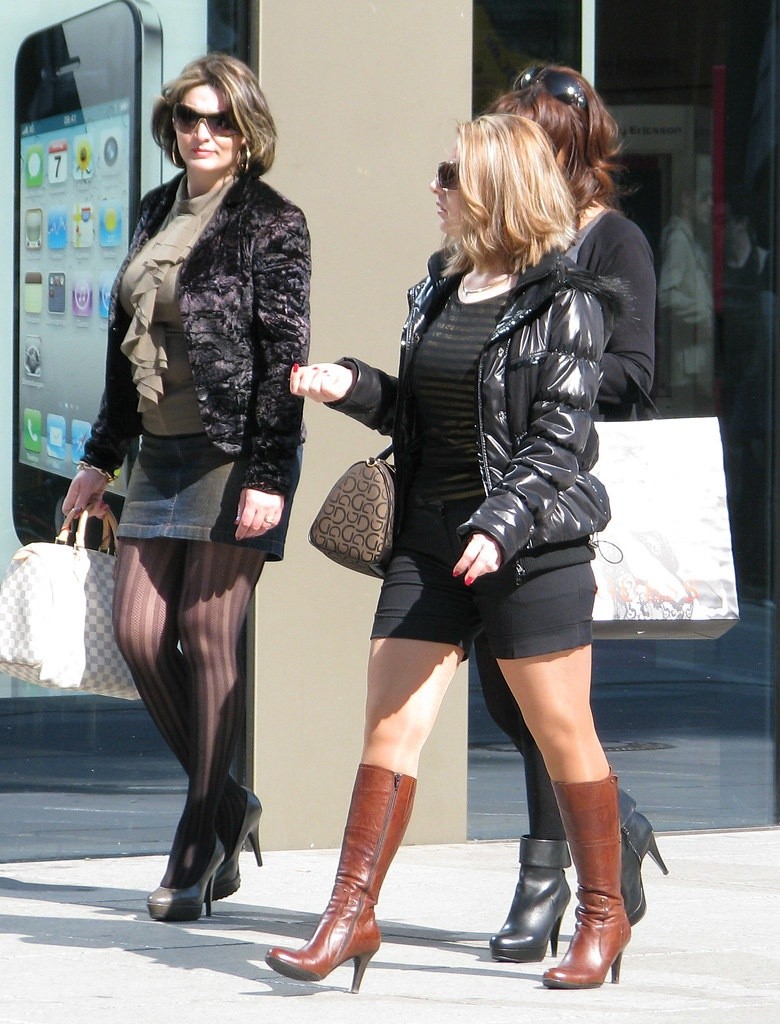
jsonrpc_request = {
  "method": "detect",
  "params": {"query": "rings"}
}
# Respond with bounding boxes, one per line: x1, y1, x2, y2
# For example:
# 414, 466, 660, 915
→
265, 516, 273, 523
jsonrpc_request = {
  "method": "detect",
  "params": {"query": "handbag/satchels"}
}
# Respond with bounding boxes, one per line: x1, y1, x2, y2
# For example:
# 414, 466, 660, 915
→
307, 443, 399, 580
660, 226, 715, 350
0, 503, 142, 701
588, 353, 741, 642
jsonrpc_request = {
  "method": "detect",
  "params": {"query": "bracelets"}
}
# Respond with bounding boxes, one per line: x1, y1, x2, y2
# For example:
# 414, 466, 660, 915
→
77, 461, 117, 482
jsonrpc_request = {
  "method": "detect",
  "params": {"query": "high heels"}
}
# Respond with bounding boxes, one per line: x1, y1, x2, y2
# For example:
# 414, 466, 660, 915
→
147, 833, 225, 922
202, 786, 263, 903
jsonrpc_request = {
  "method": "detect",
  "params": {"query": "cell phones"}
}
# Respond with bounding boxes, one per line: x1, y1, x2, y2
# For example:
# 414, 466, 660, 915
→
11, 0, 165, 550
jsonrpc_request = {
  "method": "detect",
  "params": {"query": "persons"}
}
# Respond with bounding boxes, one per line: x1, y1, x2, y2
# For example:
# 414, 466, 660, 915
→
489, 62, 668, 962
656, 170, 775, 421
262, 110, 630, 995
59, 52, 311, 921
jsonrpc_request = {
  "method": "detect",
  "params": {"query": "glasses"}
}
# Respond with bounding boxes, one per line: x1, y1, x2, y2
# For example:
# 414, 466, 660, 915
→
686, 189, 712, 203
514, 65, 590, 114
170, 102, 242, 137
436, 161, 460, 191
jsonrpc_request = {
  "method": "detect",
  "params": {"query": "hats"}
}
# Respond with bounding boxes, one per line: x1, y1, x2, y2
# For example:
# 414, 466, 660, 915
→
678, 154, 712, 184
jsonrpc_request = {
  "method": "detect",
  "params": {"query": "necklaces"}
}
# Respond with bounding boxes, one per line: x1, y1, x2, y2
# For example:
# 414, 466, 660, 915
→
461, 270, 515, 296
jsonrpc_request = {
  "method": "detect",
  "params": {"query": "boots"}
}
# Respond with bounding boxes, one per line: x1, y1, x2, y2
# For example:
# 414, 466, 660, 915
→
543, 768, 631, 989
617, 788, 670, 927
489, 835, 571, 963
265, 763, 418, 994
